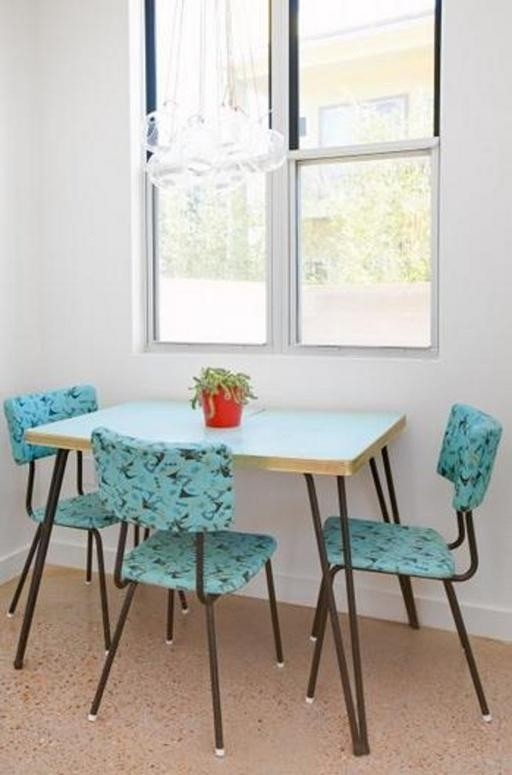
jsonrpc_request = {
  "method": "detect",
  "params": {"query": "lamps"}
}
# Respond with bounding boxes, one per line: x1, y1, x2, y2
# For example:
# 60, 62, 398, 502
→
139, 1, 288, 205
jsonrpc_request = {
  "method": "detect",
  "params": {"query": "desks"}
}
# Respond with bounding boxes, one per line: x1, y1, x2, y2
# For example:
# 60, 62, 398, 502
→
12, 400, 419, 756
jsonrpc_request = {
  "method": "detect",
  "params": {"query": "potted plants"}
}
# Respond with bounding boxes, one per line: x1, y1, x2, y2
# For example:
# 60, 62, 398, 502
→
188, 367, 258, 427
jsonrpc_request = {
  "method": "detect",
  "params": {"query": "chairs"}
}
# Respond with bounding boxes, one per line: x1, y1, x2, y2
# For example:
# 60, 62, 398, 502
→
305, 403, 503, 723
3, 384, 190, 656
90, 426, 285, 757
